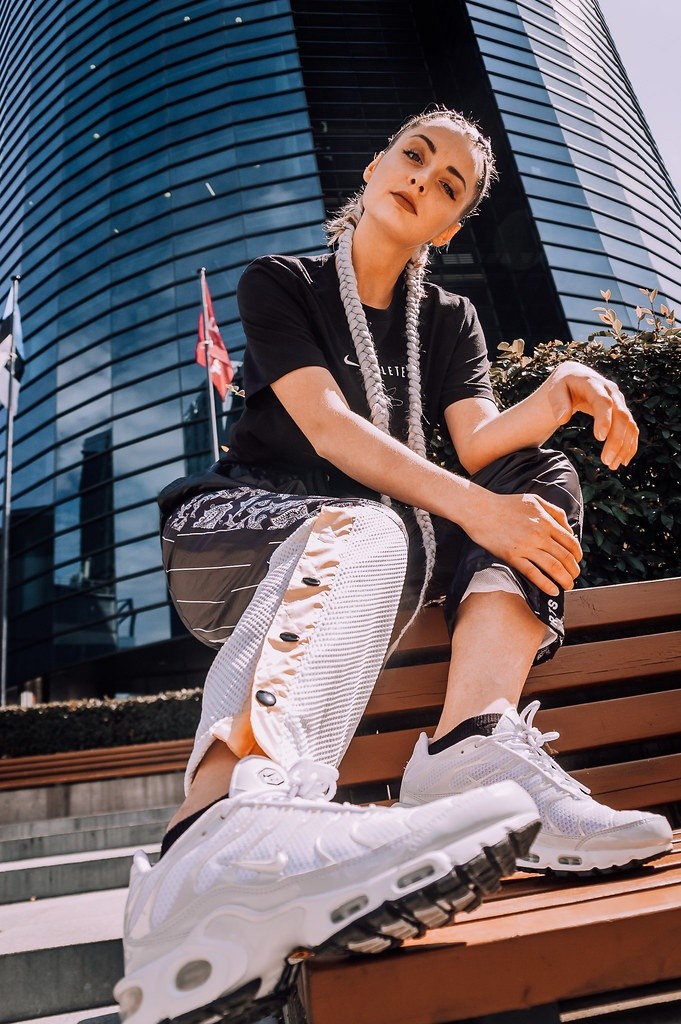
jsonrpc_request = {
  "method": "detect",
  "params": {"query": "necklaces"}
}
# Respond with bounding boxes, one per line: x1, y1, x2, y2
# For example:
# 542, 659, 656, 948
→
335, 220, 438, 683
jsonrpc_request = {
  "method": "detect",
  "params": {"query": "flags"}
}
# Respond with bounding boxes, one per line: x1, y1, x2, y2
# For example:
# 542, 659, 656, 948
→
0, 283, 25, 416
195, 273, 234, 400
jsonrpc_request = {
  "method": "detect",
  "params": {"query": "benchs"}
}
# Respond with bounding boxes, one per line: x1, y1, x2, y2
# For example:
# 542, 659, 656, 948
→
296, 576, 681, 1024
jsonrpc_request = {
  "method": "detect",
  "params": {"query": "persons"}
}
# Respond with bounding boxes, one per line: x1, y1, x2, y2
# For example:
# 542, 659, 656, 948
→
113, 103, 673, 1024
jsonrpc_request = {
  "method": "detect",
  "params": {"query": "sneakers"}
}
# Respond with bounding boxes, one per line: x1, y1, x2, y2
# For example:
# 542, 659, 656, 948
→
112, 755, 543, 1024
391, 701, 674, 880
